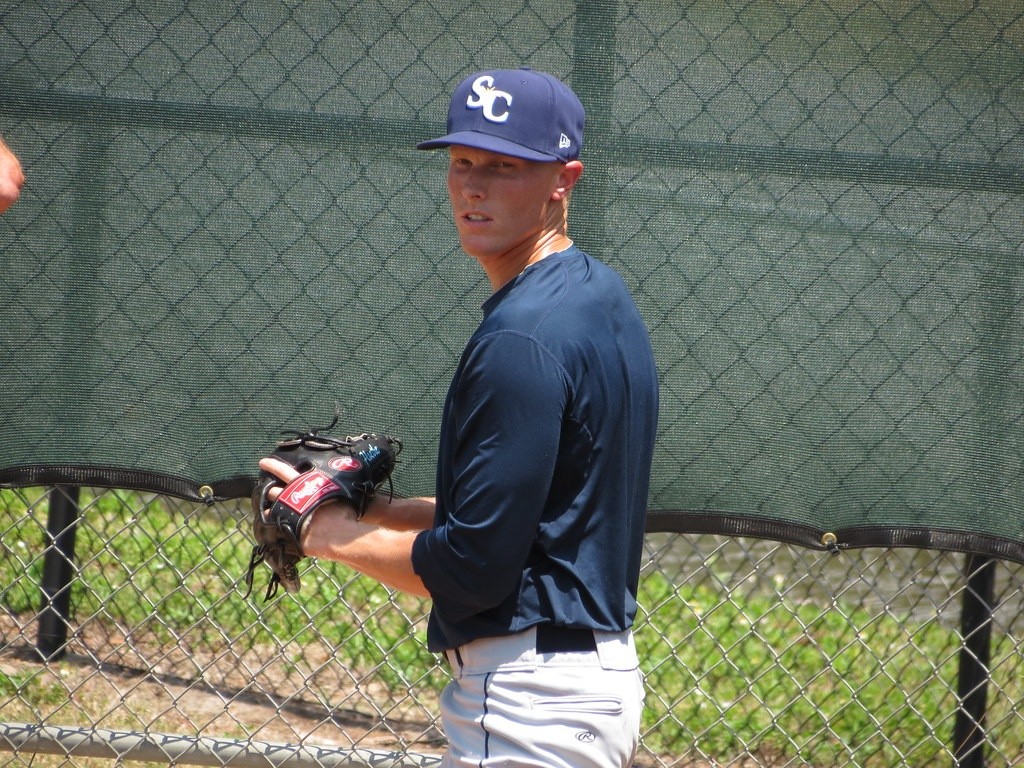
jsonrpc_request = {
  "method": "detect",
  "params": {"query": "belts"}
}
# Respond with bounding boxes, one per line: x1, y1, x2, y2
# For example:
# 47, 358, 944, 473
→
441, 625, 598, 671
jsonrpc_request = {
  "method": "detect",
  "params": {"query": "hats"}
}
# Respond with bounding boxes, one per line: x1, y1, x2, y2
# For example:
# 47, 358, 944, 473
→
417, 67, 586, 163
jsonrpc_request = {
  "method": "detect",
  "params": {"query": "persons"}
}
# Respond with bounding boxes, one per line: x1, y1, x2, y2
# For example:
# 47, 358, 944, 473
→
252, 69, 661, 767
